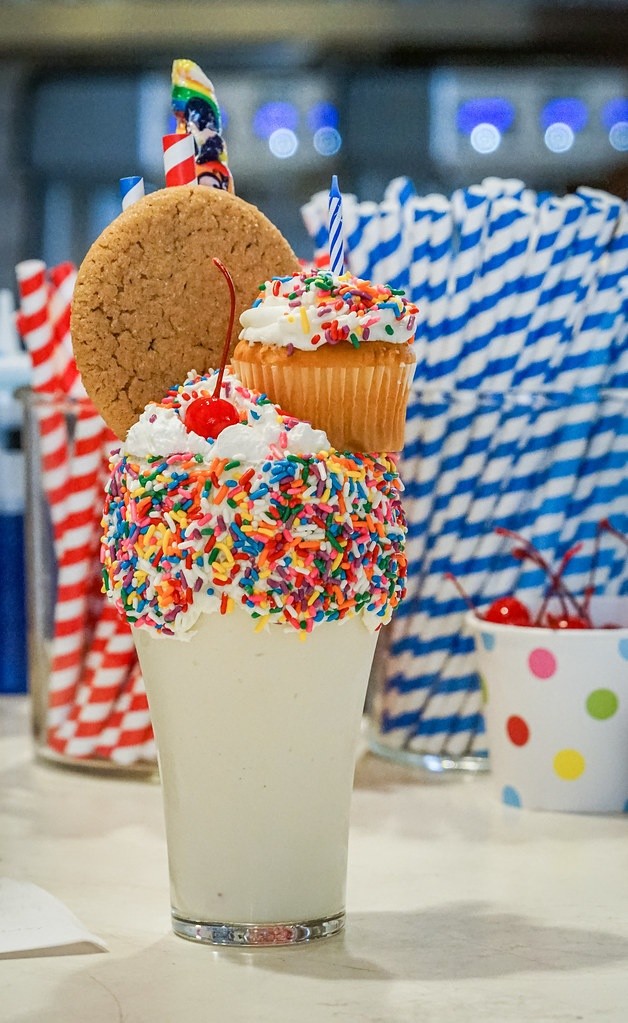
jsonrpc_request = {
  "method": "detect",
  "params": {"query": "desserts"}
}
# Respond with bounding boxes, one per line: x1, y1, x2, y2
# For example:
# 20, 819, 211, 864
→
72, 55, 417, 922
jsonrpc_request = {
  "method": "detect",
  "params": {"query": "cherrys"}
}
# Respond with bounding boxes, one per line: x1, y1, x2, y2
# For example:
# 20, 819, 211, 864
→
444, 518, 628, 630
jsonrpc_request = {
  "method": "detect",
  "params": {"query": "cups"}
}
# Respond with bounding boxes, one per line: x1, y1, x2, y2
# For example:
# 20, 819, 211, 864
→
15, 380, 628, 944
